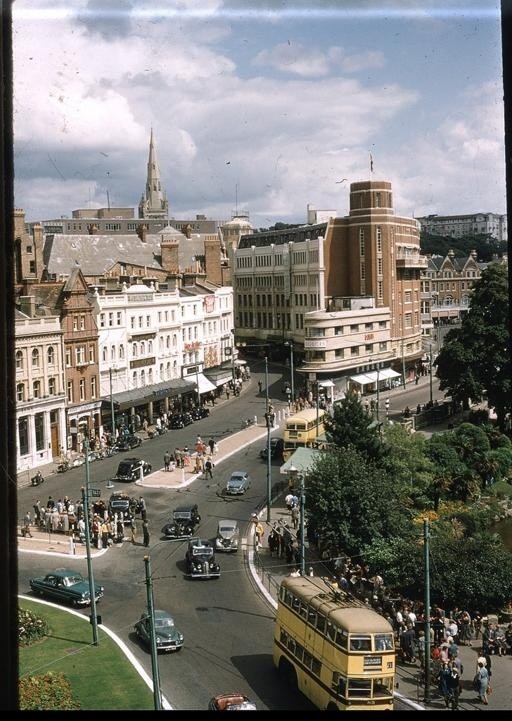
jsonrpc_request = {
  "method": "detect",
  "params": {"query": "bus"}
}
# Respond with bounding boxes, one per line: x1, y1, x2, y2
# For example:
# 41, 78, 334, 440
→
272, 572, 398, 711
283, 406, 329, 463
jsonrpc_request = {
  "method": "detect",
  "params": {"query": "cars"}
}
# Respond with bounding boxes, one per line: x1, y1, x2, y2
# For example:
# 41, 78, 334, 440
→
115, 433, 141, 451
29, 567, 104, 607
135, 609, 184, 654
107, 491, 132, 526
170, 412, 192, 429
226, 470, 251, 495
210, 691, 258, 710
262, 436, 284, 459
192, 405, 208, 420
116, 456, 151, 482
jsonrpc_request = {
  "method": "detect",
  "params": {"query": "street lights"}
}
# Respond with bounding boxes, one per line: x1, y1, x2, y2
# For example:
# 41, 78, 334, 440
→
288, 457, 307, 574
84, 440, 106, 510
284, 339, 296, 410
80, 479, 116, 646
110, 364, 121, 444
144, 536, 202, 710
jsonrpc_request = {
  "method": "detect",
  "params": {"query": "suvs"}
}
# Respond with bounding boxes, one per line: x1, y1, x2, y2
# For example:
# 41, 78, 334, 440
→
166, 503, 202, 539
185, 539, 220, 580
216, 518, 239, 552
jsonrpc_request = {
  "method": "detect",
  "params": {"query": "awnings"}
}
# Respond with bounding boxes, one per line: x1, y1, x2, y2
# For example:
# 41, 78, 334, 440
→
350, 368, 402, 385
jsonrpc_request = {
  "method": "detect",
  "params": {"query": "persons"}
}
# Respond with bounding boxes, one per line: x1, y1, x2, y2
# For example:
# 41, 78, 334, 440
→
265, 396, 274, 428
276, 553, 512, 712
162, 434, 218, 482
87, 410, 168, 451
365, 397, 390, 412
422, 365, 428, 376
22, 495, 152, 551
284, 380, 326, 406
402, 405, 420, 418
258, 380, 262, 393
434, 317, 451, 327
210, 391, 215, 408
